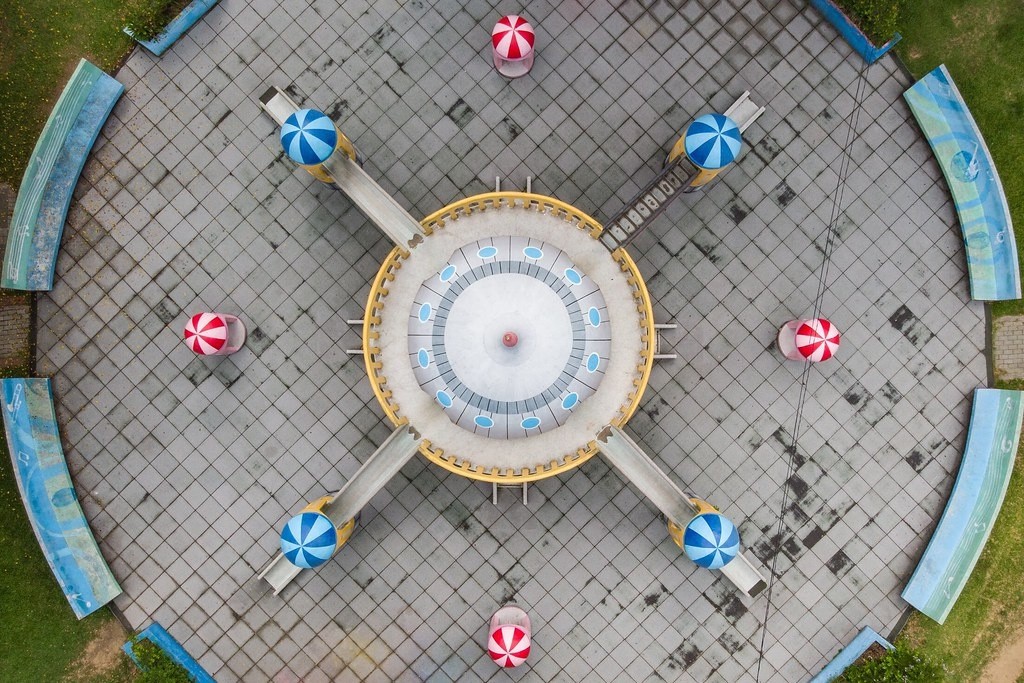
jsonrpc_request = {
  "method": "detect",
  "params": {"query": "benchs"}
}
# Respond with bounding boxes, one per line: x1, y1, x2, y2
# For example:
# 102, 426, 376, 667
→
900, 388, 1024, 625
903, 63, 1023, 301
1, 57, 125, 292
0, 377, 124, 622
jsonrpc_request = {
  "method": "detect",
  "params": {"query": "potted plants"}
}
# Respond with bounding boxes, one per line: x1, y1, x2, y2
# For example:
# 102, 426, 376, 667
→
122, 0, 218, 56
810, 0, 903, 66
